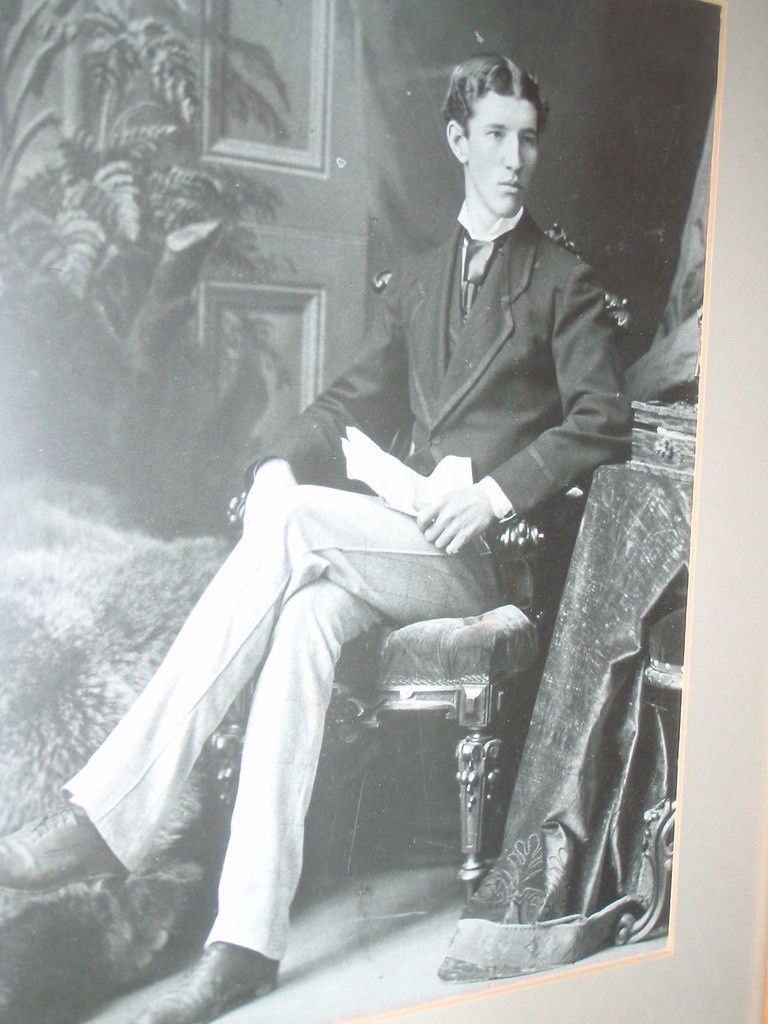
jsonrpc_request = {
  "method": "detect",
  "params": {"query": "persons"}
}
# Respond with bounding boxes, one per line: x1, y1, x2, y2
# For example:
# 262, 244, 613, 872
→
0, 53, 632, 1024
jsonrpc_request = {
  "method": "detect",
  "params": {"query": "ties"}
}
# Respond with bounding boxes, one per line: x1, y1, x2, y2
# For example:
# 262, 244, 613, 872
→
456, 218, 515, 326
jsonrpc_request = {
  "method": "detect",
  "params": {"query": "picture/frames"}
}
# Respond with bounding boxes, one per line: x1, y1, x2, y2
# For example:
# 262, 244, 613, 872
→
195, 281, 326, 416
199, 0, 335, 181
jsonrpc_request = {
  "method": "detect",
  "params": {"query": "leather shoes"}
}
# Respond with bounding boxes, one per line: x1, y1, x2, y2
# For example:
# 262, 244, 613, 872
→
131, 940, 280, 1024
0, 795, 127, 896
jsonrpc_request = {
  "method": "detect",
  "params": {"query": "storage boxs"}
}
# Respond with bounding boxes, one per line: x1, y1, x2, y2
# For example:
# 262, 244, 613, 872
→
624, 394, 699, 485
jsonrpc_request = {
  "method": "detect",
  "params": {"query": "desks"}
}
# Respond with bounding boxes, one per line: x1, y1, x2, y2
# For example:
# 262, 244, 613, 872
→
436, 461, 694, 986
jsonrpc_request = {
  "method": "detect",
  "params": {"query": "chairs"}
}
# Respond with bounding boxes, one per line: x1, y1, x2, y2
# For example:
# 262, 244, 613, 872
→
198, 220, 636, 909
